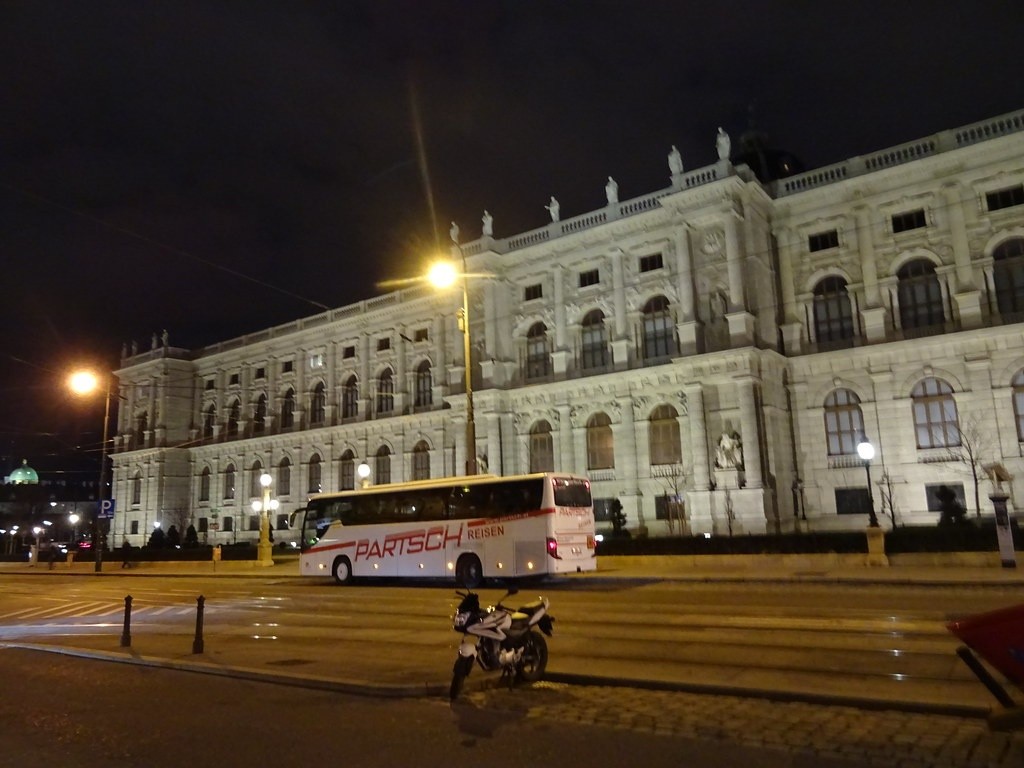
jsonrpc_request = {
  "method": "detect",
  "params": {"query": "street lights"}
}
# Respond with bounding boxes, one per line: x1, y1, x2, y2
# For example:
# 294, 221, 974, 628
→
856, 436, 888, 567
427, 237, 477, 477
357, 463, 371, 490
70, 369, 110, 572
258, 473, 274, 567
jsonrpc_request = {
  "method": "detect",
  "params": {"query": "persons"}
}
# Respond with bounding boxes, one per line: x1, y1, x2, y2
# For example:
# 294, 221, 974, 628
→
121, 329, 169, 360
448, 220, 459, 249
710, 290, 728, 318
121, 539, 132, 568
603, 175, 619, 204
714, 127, 732, 160
544, 196, 560, 222
476, 454, 489, 474
668, 146, 683, 175
714, 431, 743, 470
480, 210, 494, 237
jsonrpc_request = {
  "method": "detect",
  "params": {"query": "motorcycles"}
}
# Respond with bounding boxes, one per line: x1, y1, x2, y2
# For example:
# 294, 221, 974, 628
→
448, 567, 555, 699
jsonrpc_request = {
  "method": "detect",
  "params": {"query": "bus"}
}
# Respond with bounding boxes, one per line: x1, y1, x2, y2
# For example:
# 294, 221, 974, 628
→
289, 471, 597, 586
